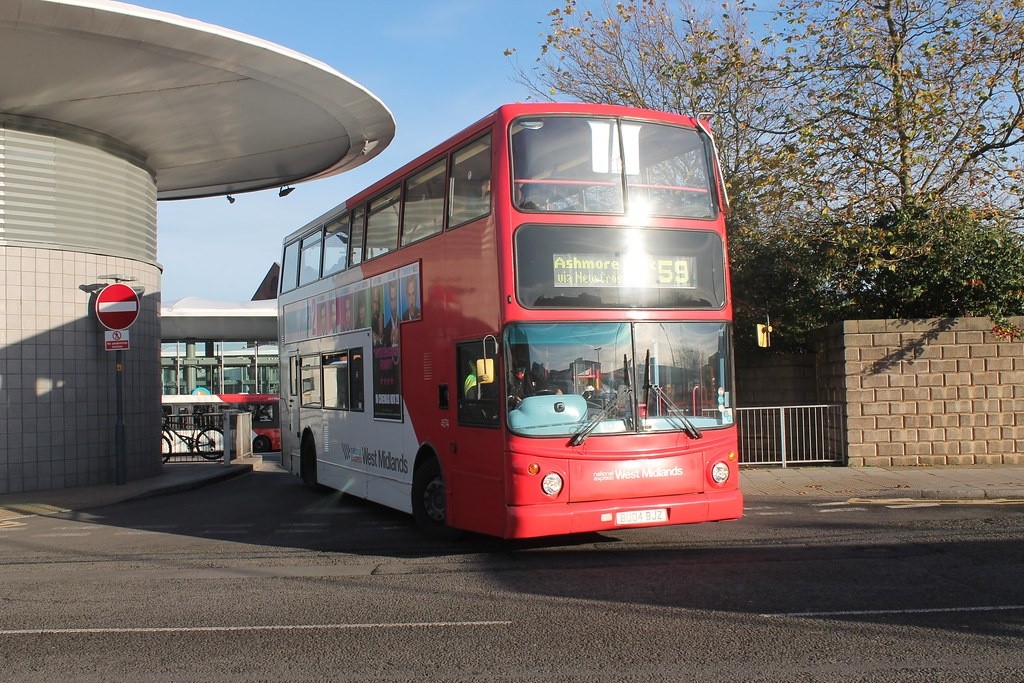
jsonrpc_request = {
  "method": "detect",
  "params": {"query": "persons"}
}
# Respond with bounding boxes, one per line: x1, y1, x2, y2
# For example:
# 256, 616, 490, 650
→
506, 354, 563, 407
319, 275, 420, 349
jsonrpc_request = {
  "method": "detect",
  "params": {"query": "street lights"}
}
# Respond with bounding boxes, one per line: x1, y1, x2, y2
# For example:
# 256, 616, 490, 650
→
95, 273, 138, 486
594, 348, 602, 363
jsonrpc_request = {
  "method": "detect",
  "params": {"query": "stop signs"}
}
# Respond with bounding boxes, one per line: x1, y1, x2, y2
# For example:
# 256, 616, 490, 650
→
95, 282, 141, 332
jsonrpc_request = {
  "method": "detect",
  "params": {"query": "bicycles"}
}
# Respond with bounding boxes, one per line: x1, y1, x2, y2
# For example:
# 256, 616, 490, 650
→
161, 412, 225, 464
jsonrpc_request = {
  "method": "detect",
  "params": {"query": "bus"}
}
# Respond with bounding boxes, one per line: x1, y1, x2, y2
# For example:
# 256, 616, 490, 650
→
161, 393, 281, 455
277, 102, 774, 546
